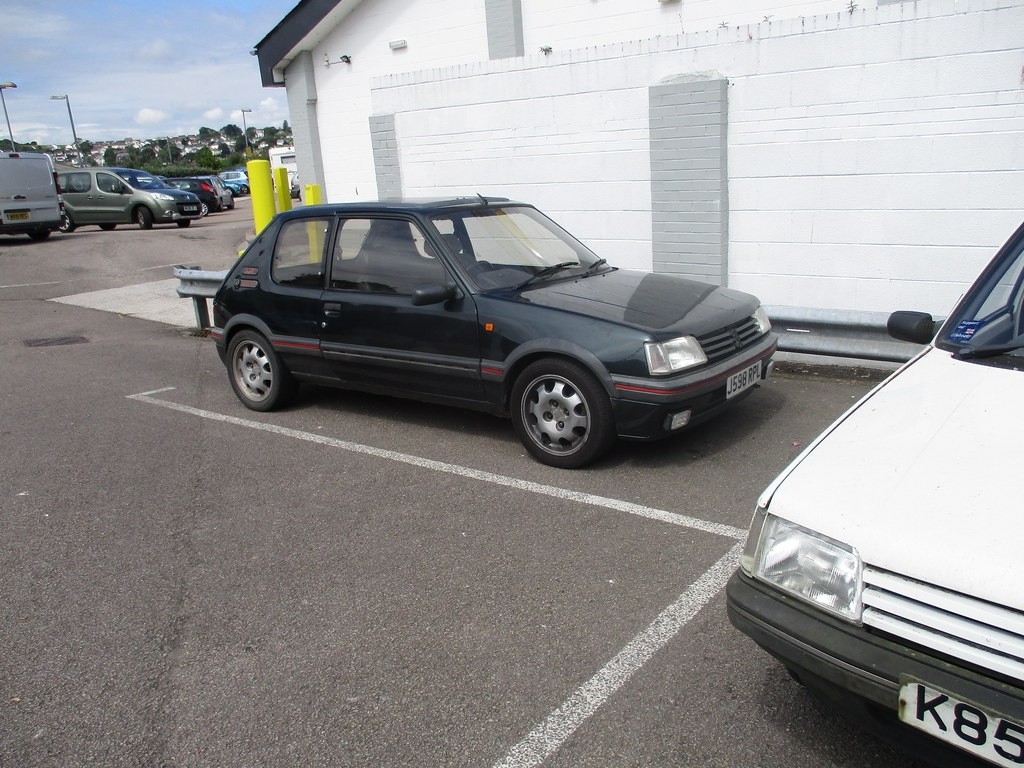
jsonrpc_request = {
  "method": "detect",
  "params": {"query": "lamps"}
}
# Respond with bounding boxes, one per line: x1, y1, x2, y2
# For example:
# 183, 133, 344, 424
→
388, 39, 407, 50
340, 55, 350, 63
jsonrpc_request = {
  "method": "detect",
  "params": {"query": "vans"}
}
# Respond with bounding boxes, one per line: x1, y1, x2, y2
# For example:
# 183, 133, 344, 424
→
0, 151, 63, 240
54, 167, 203, 235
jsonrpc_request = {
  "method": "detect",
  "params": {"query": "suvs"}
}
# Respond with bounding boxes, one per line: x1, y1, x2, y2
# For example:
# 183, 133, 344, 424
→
142, 178, 217, 216
290, 174, 300, 200
218, 171, 250, 194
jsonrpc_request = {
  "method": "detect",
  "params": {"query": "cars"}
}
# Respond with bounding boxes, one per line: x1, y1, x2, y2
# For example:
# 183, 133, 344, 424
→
725, 225, 1024, 768
181, 174, 235, 211
223, 182, 245, 197
205, 194, 778, 470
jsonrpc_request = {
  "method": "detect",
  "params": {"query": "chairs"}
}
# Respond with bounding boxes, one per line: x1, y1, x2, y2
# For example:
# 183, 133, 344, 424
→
353, 232, 423, 293
424, 234, 477, 287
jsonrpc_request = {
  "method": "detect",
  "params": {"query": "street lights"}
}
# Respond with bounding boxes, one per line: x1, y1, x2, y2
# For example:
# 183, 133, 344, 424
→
50, 94, 82, 168
0, 82, 17, 152
242, 109, 252, 161
